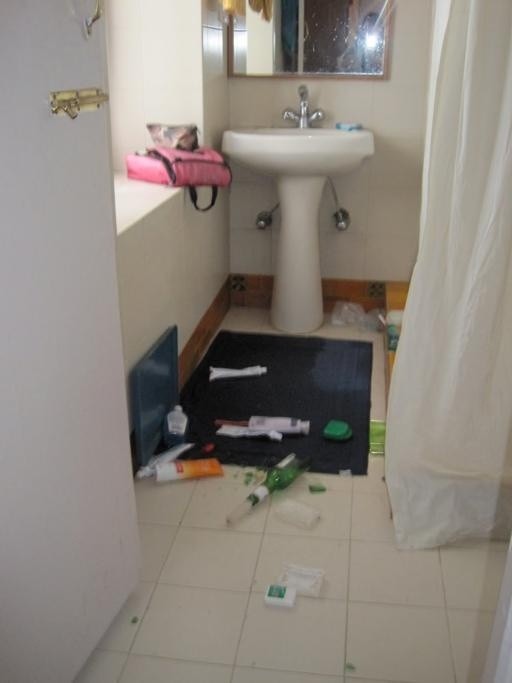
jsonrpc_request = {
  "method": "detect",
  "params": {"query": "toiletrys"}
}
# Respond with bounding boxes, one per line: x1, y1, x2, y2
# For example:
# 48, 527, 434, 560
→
249, 416, 310, 435
167, 404, 189, 437
155, 459, 224, 482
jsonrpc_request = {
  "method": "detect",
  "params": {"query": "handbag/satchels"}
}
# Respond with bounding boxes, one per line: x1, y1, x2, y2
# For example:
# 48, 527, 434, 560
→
124, 146, 233, 190
145, 121, 198, 151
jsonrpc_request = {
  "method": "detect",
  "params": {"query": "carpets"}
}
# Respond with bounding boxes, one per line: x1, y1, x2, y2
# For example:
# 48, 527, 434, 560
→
154, 330, 375, 475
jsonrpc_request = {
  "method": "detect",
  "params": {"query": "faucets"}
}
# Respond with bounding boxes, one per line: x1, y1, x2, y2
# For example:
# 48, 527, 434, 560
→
297, 84, 310, 116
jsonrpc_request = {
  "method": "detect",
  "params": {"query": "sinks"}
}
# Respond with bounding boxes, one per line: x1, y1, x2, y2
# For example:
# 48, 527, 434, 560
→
221, 127, 374, 176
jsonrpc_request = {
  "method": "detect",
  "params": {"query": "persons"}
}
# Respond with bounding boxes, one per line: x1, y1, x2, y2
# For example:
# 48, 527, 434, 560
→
337, 12, 383, 70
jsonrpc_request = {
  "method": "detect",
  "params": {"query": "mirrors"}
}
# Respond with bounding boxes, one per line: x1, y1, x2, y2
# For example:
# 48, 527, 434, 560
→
225, 0, 391, 78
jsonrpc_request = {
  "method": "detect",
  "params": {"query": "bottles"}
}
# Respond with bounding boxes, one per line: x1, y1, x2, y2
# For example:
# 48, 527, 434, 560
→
225, 449, 312, 525
386, 313, 400, 353
165, 403, 190, 447
